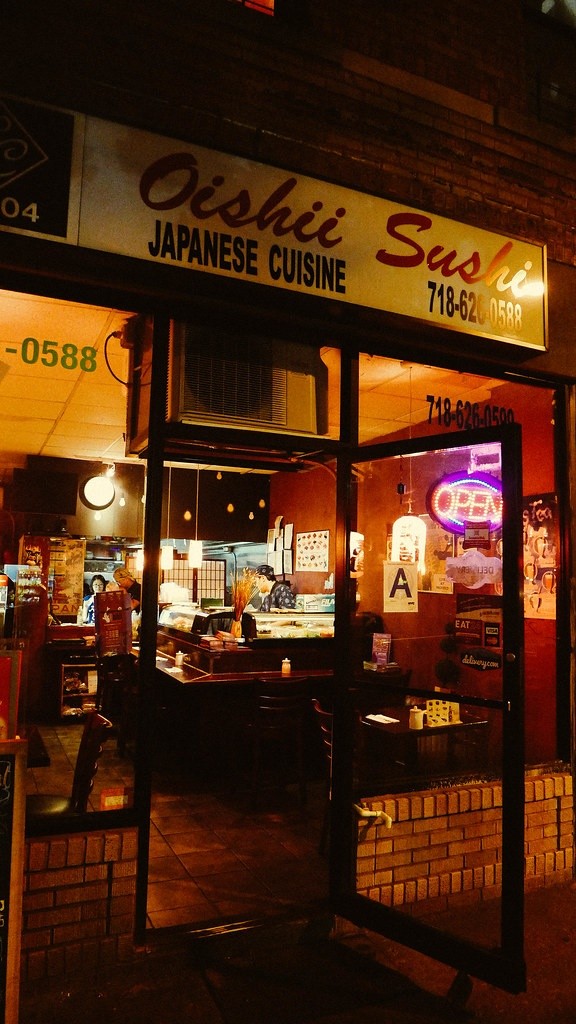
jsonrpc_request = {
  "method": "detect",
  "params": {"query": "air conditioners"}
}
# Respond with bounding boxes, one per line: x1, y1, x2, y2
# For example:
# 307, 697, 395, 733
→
134, 316, 317, 456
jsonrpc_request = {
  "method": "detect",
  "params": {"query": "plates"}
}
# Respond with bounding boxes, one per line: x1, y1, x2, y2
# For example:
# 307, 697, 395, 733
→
96, 556, 113, 559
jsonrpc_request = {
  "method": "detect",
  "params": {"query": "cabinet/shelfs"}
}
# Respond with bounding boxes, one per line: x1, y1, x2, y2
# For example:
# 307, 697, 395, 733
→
57, 662, 96, 719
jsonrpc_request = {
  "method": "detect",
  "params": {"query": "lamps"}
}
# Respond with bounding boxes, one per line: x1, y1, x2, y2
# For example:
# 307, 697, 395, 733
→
135, 465, 203, 572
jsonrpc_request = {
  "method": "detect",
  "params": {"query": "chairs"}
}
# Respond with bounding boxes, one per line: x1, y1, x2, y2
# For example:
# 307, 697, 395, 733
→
26, 713, 114, 817
234, 675, 310, 812
311, 699, 412, 859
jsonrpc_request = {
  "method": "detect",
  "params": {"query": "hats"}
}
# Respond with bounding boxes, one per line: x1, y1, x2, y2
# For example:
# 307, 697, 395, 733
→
255, 565, 274, 575
113, 568, 130, 579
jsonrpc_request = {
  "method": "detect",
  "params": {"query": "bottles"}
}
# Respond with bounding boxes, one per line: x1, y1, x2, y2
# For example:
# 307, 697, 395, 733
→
175, 651, 183, 665
18, 572, 45, 585
282, 658, 291, 673
409, 706, 423, 729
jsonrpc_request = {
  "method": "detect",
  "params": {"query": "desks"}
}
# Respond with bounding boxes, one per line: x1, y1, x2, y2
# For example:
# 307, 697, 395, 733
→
18, 726, 50, 768
357, 703, 487, 792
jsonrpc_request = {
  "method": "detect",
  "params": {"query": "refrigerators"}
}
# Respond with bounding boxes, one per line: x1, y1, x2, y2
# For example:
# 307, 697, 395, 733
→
0, 572, 15, 637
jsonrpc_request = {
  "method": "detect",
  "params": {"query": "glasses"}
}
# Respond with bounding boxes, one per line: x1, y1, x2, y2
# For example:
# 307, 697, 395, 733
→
92, 583, 104, 588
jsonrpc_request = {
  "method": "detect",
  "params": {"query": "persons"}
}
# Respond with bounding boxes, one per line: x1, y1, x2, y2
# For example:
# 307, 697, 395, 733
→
82, 575, 106, 625
254, 564, 295, 613
114, 567, 142, 615
